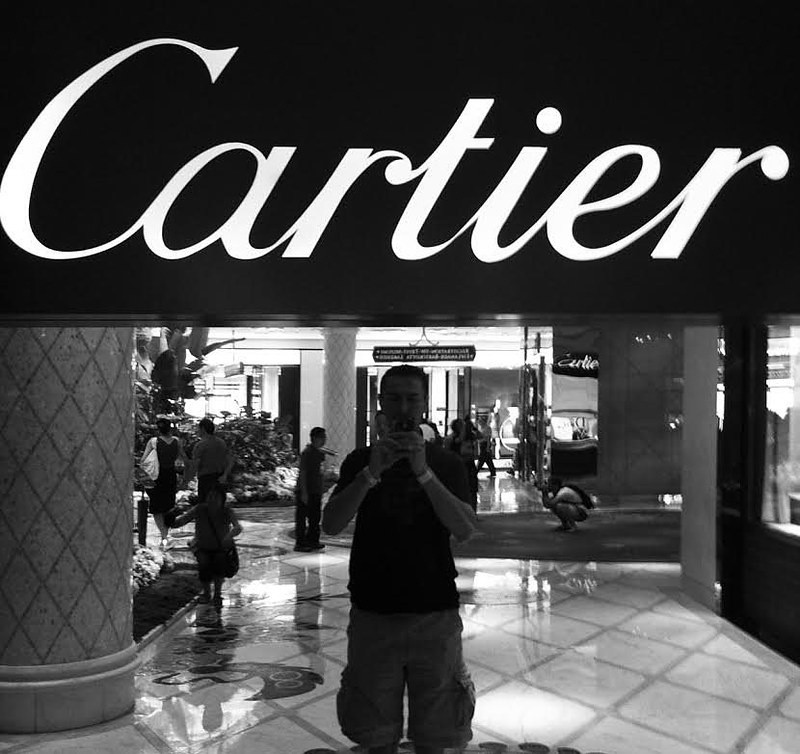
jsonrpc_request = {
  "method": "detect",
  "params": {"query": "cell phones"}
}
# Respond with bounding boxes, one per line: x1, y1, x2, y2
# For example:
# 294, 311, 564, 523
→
393, 420, 414, 432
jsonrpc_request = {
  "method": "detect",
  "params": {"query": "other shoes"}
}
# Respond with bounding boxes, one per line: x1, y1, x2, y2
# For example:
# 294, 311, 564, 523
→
487, 472, 497, 478
507, 469, 515, 477
294, 540, 326, 551
197, 593, 222, 606
555, 525, 579, 533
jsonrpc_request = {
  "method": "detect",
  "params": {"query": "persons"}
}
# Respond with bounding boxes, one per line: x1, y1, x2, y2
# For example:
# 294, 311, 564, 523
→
323, 366, 479, 747
293, 427, 326, 552
141, 417, 243, 605
375, 400, 549, 489
537, 476, 589, 531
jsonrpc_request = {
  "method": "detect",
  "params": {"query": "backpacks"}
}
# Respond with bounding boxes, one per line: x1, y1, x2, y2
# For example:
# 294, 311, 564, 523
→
566, 483, 595, 509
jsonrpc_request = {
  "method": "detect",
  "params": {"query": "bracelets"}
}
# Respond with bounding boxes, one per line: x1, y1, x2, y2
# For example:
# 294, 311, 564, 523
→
363, 466, 380, 488
417, 468, 435, 484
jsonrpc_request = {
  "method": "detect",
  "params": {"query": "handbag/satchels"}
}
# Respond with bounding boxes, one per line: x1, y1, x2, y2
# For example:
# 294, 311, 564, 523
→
217, 539, 239, 577
140, 437, 160, 480
